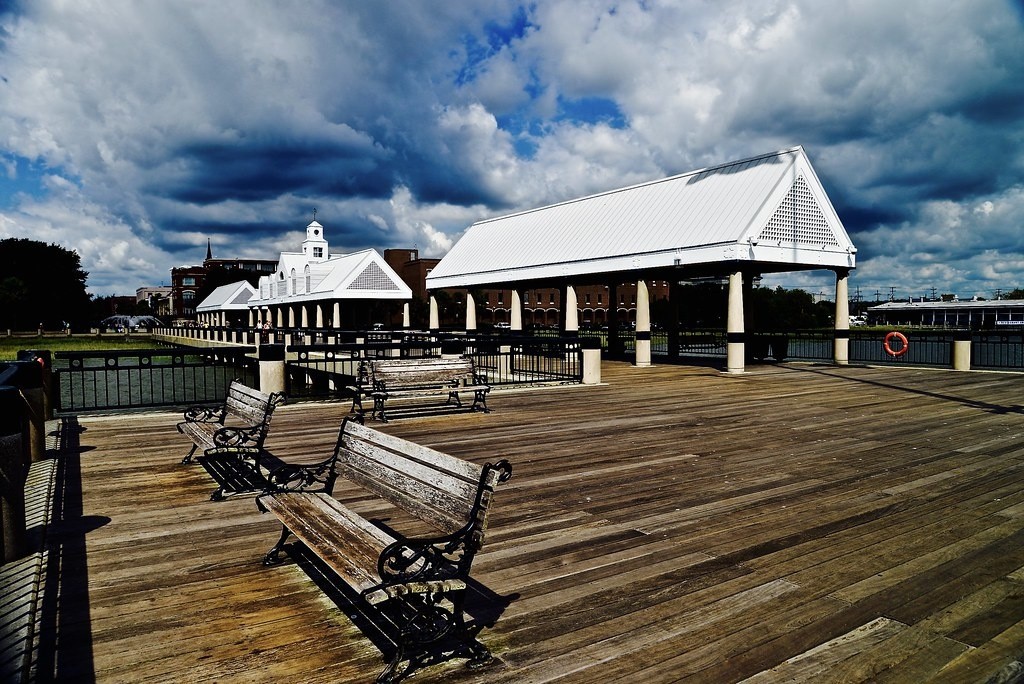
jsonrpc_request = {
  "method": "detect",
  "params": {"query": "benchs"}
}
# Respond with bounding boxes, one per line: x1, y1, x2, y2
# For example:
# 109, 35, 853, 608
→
175, 377, 289, 502
254, 415, 513, 684
341, 356, 496, 422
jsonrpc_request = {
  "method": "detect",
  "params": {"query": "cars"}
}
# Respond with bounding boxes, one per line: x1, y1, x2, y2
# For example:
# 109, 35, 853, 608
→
101, 314, 165, 329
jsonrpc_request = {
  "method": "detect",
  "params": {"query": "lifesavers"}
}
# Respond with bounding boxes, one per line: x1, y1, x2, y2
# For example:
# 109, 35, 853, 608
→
884, 331, 909, 356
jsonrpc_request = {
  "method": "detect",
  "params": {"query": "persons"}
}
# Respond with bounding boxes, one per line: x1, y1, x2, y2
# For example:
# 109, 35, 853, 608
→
61, 321, 68, 334
38, 323, 45, 335
256, 320, 274, 342
101, 316, 129, 333
225, 321, 230, 328
236, 319, 243, 328
177, 320, 208, 327
